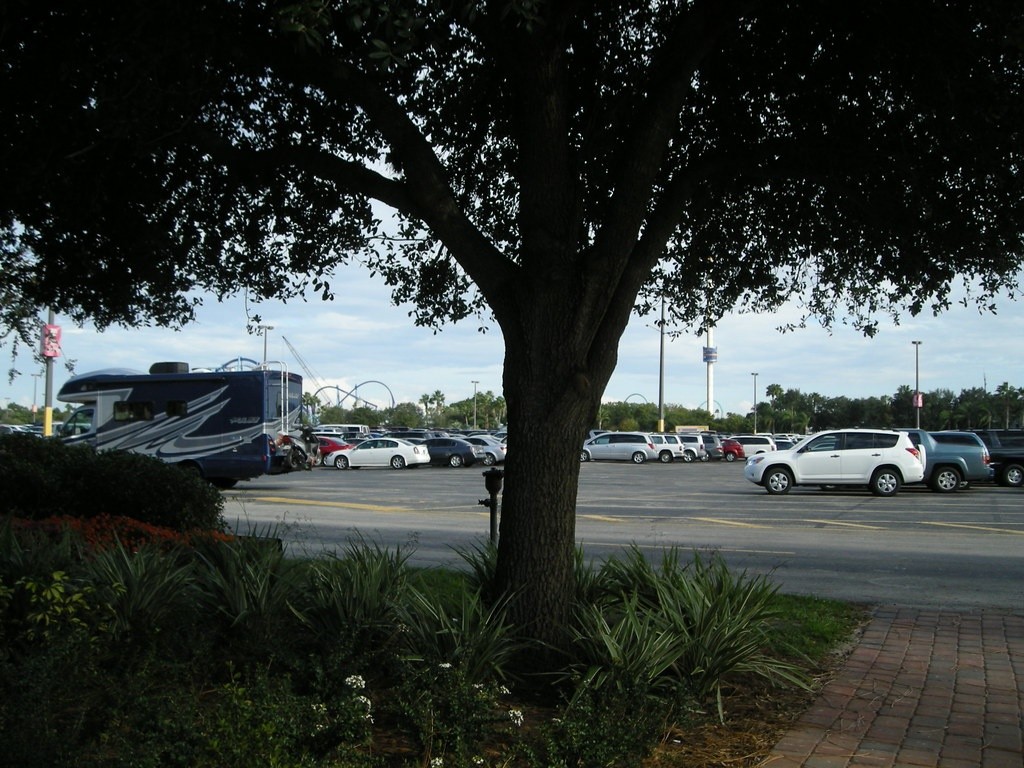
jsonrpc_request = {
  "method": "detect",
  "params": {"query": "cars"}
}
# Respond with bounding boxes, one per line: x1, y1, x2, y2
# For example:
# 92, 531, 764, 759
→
421, 428, 507, 468
320, 437, 432, 469
579, 432, 659, 464
0, 421, 91, 438
650, 431, 811, 464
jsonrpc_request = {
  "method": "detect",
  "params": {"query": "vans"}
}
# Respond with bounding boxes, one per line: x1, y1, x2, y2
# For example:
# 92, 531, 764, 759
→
311, 424, 449, 438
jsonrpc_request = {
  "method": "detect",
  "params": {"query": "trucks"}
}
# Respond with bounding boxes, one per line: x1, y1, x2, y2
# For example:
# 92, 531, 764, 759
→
54, 362, 302, 489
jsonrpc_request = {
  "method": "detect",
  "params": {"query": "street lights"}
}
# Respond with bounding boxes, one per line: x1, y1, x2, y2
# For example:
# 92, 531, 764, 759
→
469, 379, 479, 424
912, 341, 923, 428
751, 373, 758, 433
257, 324, 276, 368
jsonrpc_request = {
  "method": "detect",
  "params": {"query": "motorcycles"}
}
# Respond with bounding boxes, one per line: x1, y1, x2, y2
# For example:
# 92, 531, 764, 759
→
277, 426, 322, 471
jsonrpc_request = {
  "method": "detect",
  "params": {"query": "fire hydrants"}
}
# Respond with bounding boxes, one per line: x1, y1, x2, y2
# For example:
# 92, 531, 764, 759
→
478, 466, 504, 545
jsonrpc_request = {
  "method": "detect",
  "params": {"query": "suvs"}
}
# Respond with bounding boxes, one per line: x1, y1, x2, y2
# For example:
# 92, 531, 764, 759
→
744, 428, 927, 497
893, 428, 1024, 493
